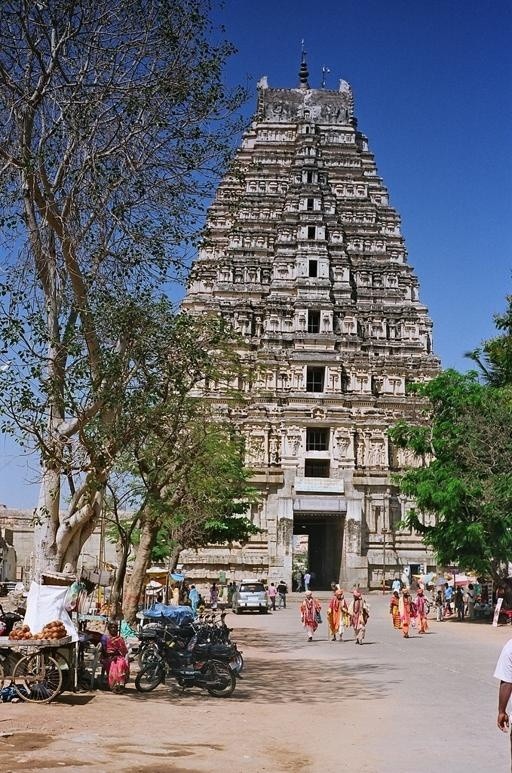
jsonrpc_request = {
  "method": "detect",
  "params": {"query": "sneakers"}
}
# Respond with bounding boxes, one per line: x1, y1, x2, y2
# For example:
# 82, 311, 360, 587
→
113, 685, 128, 695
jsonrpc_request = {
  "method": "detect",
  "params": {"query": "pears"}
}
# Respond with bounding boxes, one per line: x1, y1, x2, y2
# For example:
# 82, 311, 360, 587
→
8, 621, 67, 640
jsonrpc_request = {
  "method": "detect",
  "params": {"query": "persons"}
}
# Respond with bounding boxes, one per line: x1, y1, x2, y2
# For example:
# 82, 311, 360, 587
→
210, 583, 220, 595
268, 579, 288, 611
188, 585, 200, 618
100, 621, 130, 695
326, 590, 349, 641
296, 571, 302, 592
347, 590, 369, 645
493, 639, 512, 773
299, 591, 323, 642
388, 573, 505, 638
221, 581, 237, 613
209, 587, 219, 611
303, 570, 311, 591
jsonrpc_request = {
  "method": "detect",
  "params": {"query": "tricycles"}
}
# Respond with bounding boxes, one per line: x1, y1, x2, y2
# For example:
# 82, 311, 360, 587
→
0, 632, 76, 704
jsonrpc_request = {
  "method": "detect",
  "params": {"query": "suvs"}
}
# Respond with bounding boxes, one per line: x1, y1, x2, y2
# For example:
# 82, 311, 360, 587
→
233, 579, 269, 618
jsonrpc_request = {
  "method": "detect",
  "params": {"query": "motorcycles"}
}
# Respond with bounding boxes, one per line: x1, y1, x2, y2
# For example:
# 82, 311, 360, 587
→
133, 606, 244, 696
0, 605, 99, 687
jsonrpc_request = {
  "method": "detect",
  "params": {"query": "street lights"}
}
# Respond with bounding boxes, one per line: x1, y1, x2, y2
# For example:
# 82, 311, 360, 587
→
365, 494, 396, 595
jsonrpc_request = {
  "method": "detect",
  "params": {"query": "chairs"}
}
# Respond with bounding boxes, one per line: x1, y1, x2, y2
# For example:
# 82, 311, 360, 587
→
78, 644, 106, 691
